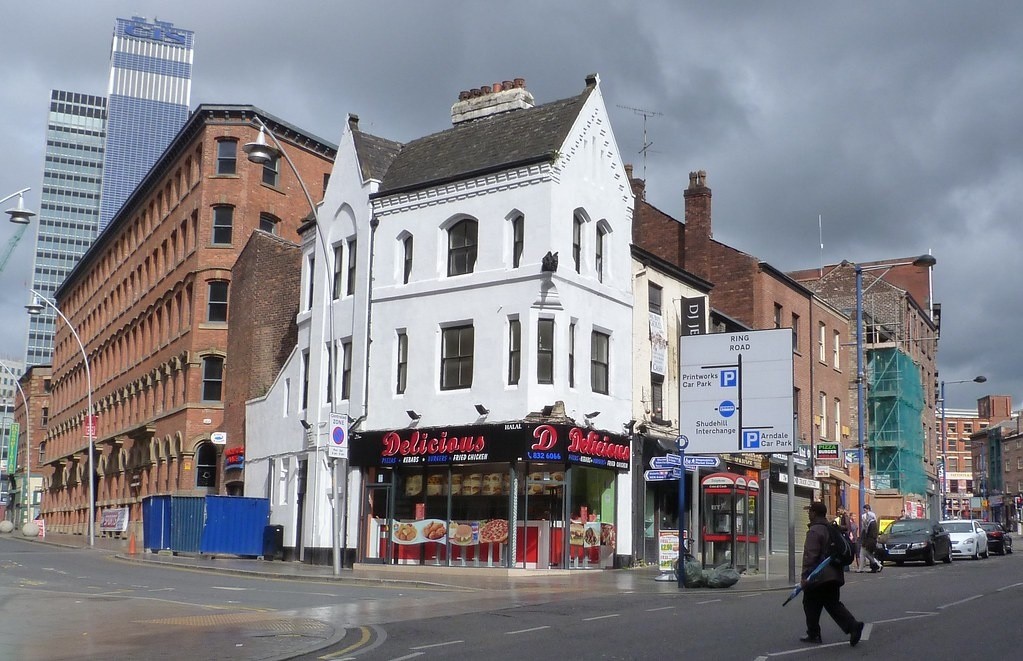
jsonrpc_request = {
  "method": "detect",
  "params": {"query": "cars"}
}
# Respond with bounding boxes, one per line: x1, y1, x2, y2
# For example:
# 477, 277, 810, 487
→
978, 522, 1013, 556
939, 520, 989, 561
876, 517, 952, 566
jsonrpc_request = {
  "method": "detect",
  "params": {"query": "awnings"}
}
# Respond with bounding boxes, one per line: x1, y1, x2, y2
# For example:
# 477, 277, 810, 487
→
830, 470, 876, 494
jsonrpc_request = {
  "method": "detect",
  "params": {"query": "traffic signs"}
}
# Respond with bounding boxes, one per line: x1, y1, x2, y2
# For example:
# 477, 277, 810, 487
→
650, 457, 678, 468
684, 456, 720, 467
666, 453, 682, 466
644, 469, 678, 481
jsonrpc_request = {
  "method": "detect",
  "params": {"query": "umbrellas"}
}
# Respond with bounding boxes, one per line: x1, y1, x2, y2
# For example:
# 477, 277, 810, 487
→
783, 556, 832, 606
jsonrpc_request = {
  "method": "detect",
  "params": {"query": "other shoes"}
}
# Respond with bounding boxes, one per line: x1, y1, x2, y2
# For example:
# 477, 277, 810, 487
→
855, 570, 864, 573
878, 565, 883, 572
800, 634, 822, 643
868, 570, 876, 573
850, 621, 864, 646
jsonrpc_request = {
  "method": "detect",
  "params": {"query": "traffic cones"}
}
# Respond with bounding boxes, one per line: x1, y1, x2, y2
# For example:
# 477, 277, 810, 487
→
129, 532, 136, 554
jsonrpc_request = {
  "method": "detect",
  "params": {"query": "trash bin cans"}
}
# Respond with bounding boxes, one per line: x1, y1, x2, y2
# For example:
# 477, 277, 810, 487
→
263, 525, 284, 561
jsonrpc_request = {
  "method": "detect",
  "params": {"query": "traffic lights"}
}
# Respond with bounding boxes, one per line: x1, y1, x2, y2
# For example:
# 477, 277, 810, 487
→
1014, 497, 1023, 510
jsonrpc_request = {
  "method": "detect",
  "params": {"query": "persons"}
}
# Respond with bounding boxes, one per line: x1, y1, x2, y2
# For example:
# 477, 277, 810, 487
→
898, 510, 910, 519
835, 504, 883, 573
798, 503, 864, 646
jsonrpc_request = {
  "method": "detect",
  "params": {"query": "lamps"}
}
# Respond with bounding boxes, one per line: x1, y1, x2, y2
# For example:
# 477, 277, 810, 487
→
586, 412, 600, 417
638, 425, 647, 434
354, 433, 362, 439
301, 420, 314, 429
625, 420, 636, 430
407, 411, 422, 420
474, 405, 490, 416
542, 406, 554, 417
347, 414, 357, 424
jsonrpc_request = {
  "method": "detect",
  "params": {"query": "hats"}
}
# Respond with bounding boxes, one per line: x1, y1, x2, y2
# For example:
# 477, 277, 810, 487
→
803, 501, 826, 516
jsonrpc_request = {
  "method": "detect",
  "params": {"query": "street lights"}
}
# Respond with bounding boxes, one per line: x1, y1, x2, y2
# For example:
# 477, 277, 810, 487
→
22, 286, 95, 547
0, 397, 8, 461
851, 255, 937, 539
958, 437, 987, 518
242, 112, 345, 578
940, 376, 987, 518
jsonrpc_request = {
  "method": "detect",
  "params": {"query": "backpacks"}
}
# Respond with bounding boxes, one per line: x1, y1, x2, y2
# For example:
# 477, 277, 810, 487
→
814, 521, 854, 566
